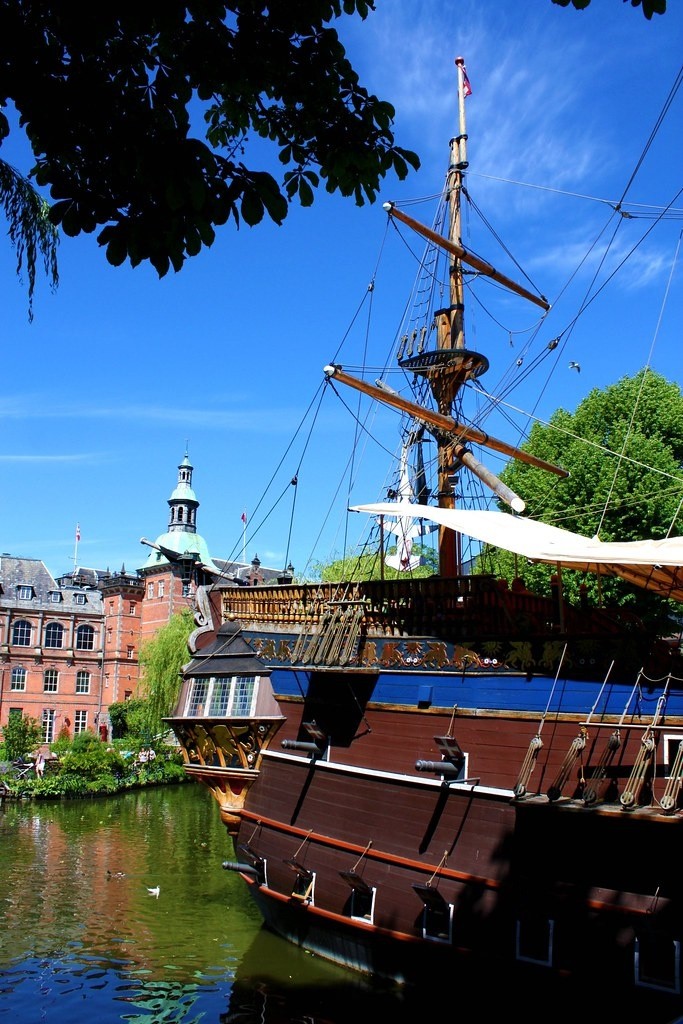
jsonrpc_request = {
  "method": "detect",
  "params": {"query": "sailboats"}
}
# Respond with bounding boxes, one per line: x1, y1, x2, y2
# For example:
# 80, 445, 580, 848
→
140, 56, 683, 1024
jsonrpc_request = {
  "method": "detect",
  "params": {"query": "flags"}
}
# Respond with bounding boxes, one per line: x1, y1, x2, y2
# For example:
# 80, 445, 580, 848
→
457, 66, 472, 99
76, 521, 81, 541
241, 513, 247, 524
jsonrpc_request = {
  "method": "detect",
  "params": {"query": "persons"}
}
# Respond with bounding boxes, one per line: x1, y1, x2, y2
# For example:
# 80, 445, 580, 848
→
496, 577, 536, 608
35, 749, 46, 779
123, 746, 156, 769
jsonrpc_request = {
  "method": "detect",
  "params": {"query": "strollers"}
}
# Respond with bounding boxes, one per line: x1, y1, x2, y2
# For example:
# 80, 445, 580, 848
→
12, 757, 35, 779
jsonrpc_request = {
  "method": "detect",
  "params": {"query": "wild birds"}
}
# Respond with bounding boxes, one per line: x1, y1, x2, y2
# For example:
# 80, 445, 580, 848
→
569, 361, 580, 373
